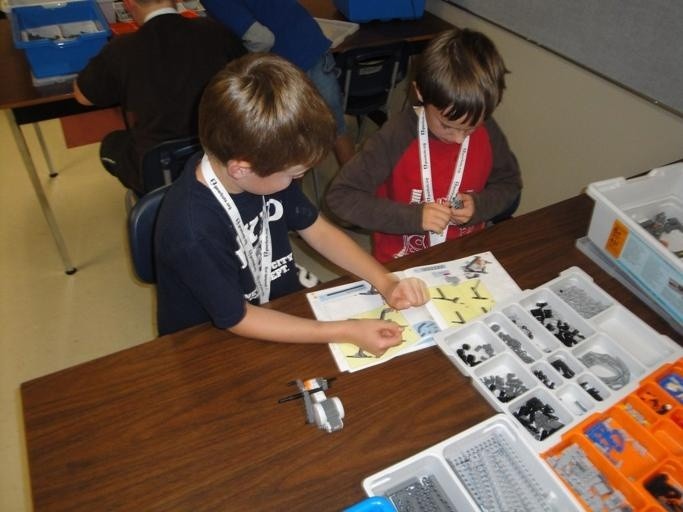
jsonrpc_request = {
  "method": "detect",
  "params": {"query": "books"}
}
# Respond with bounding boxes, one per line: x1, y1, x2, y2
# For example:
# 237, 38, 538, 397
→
305, 250, 522, 373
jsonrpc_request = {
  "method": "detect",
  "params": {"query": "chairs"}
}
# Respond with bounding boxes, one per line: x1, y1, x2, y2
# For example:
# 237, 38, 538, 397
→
142, 137, 204, 185
346, 37, 400, 136
128, 183, 172, 284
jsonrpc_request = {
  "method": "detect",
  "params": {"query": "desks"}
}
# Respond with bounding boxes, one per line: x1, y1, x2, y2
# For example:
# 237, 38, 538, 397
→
19, 158, 683, 512
0, 0, 119, 274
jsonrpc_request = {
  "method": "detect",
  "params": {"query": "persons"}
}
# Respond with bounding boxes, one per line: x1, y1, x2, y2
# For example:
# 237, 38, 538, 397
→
152, 52, 431, 358
198, 0, 354, 168
326, 26, 523, 264
72, 0, 248, 199
